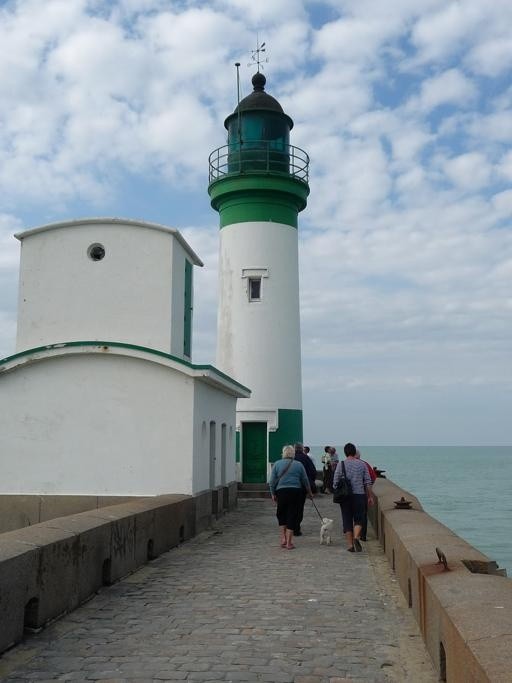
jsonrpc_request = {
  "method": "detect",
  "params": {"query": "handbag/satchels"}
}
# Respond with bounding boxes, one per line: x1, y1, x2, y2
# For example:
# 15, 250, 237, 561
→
332, 460, 353, 503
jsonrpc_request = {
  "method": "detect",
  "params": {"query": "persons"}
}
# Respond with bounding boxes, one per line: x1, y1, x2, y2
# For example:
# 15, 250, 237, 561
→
267, 441, 378, 551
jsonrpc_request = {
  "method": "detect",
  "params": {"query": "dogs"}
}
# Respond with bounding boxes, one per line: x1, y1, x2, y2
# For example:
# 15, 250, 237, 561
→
319, 517, 335, 546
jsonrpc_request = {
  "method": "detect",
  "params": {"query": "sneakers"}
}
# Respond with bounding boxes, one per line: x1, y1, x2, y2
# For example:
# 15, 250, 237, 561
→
346, 532, 367, 552
279, 525, 303, 550
